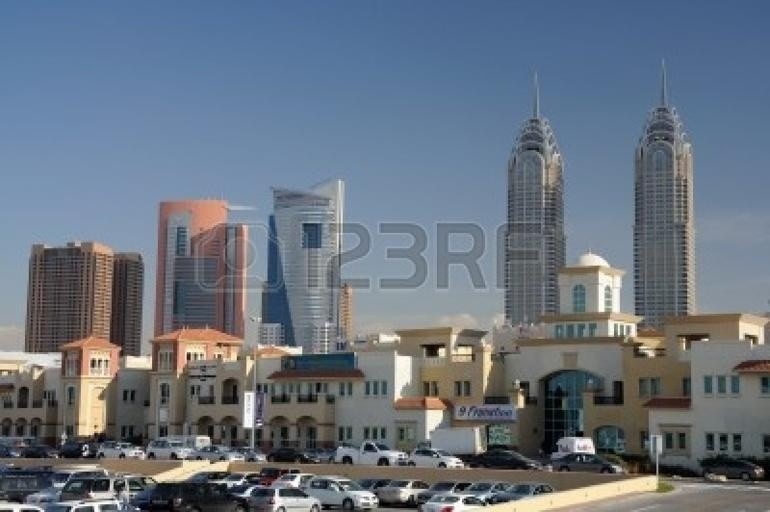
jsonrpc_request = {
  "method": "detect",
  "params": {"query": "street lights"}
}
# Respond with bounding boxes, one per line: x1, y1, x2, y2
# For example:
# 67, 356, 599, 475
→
250, 316, 256, 450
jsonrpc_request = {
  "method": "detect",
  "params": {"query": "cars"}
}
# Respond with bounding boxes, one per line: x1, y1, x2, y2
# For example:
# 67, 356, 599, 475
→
0, 467, 156, 511
359, 479, 554, 512
552, 452, 615, 474
470, 448, 541, 470
131, 470, 380, 512
704, 458, 764, 482
